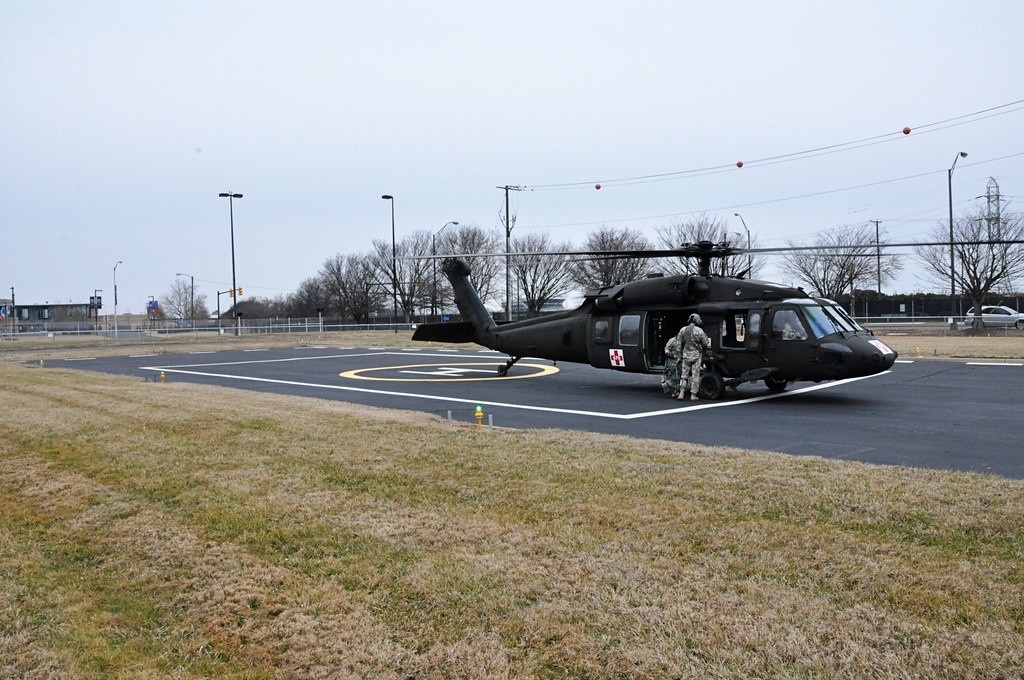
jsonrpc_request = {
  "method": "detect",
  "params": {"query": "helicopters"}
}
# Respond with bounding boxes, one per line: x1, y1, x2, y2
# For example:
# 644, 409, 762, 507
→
380, 240, 1023, 400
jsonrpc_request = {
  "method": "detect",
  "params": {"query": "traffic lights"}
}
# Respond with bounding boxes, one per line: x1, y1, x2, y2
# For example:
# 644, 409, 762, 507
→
229, 288, 233, 298
239, 286, 242, 296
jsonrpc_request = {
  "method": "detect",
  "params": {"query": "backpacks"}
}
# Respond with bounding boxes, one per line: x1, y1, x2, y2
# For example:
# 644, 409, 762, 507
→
661, 376, 674, 394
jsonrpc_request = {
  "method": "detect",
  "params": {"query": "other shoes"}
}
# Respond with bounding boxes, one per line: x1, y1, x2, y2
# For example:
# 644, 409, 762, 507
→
672, 390, 679, 397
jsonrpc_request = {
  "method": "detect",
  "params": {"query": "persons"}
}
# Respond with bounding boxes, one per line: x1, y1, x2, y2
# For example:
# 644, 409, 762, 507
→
678, 314, 709, 399
662, 336, 680, 397
783, 314, 805, 339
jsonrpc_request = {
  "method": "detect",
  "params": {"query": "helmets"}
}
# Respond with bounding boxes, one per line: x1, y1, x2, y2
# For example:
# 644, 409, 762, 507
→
687, 313, 702, 325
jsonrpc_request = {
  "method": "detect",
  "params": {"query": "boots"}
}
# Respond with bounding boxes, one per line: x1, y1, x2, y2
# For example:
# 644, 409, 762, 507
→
678, 389, 685, 400
690, 393, 699, 400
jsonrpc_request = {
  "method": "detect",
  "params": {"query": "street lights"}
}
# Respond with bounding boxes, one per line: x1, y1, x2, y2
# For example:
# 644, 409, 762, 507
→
965, 304, 1024, 329
734, 214, 751, 279
433, 221, 461, 315
723, 232, 741, 267
9, 304, 97, 333
95, 290, 104, 331
382, 194, 398, 331
948, 152, 968, 330
219, 193, 244, 335
148, 296, 155, 330
114, 261, 123, 338
177, 273, 193, 333
10, 287, 14, 332
